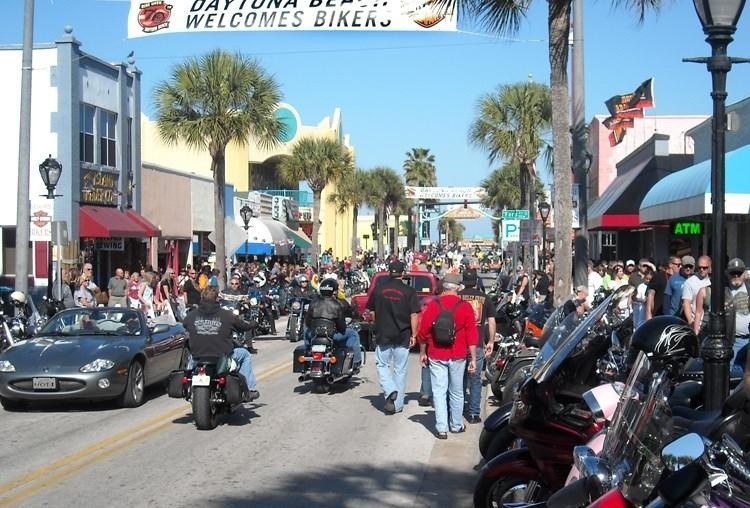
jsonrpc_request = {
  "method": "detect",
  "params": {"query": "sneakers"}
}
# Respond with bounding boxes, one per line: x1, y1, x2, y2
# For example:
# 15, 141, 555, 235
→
354, 362, 361, 370
245, 391, 260, 402
469, 415, 482, 424
384, 391, 398, 412
271, 330, 277, 335
451, 423, 466, 433
247, 347, 257, 354
438, 432, 448, 439
420, 396, 430, 406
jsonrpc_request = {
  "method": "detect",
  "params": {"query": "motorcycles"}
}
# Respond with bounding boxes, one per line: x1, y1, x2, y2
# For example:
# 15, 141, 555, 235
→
0, 286, 37, 353
291, 312, 367, 393
27, 294, 70, 338
220, 273, 367, 352
167, 319, 260, 429
424, 247, 501, 275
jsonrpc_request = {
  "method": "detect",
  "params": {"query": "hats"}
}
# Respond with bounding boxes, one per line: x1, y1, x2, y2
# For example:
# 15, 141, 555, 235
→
726, 257, 746, 273
389, 260, 406, 273
443, 272, 463, 285
576, 255, 696, 292
463, 270, 477, 286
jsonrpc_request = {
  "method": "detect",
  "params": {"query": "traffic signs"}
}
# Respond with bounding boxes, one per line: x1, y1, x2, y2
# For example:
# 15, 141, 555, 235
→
501, 209, 531, 222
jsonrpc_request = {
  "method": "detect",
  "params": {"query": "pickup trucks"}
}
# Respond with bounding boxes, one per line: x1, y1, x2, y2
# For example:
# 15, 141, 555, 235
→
353, 266, 439, 352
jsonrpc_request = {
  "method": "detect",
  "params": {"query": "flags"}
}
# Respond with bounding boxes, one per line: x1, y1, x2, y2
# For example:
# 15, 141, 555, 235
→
605, 93, 644, 117
602, 117, 634, 129
608, 128, 626, 148
623, 79, 653, 110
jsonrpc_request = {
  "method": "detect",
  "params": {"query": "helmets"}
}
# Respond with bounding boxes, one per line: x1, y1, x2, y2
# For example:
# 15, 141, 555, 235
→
9, 291, 27, 306
319, 279, 335, 293
327, 277, 339, 290
625, 315, 699, 362
299, 275, 308, 283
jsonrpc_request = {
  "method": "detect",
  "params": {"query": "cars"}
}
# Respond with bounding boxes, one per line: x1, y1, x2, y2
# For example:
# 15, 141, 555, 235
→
411, 250, 427, 265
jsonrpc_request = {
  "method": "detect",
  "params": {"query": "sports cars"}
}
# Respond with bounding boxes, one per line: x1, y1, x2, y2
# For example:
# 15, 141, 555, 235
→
0, 304, 191, 411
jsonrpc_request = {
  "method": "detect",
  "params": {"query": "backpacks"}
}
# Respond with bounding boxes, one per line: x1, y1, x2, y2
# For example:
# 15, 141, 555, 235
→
431, 295, 465, 348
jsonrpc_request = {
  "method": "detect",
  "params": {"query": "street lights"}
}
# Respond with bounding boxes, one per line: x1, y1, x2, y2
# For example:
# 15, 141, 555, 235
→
237, 201, 258, 265
678, 2, 749, 449
538, 201, 550, 278
36, 152, 67, 314
